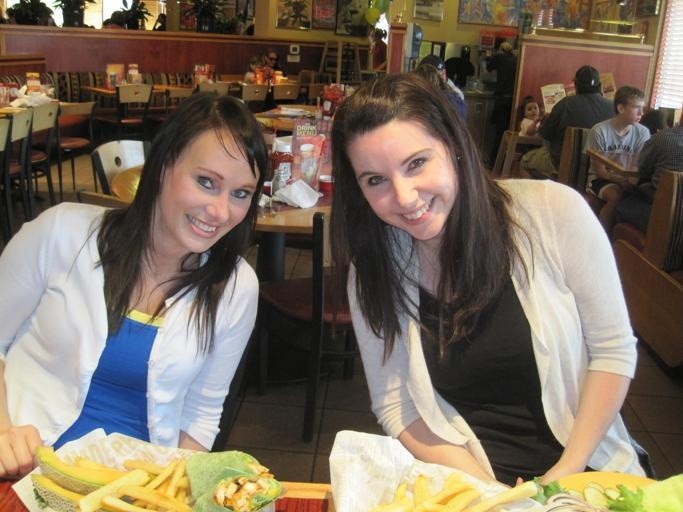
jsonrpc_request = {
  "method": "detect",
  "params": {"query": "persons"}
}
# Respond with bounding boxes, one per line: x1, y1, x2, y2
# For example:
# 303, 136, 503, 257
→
244, 49, 286, 84
372, 27, 387, 72
583, 86, 649, 239
518, 96, 542, 149
329, 76, 650, 485
484, 42, 517, 165
152, 12, 168, 30
518, 64, 617, 179
0, 8, 16, 23
415, 65, 465, 123
605, 112, 682, 242
419, 55, 465, 103
0, 92, 268, 482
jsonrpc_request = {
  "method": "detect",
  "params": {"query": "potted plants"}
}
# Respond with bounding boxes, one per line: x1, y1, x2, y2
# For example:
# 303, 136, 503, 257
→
54, 0, 95, 27
176, 0, 235, 33
116, 0, 154, 29
6, 0, 54, 25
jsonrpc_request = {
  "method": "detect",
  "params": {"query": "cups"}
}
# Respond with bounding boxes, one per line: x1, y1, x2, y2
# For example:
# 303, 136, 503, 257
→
324, 100, 332, 111
110, 71, 117, 87
519, 12, 532, 35
319, 174, 336, 192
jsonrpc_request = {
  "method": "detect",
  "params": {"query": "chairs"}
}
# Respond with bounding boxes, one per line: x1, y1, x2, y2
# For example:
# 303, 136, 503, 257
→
491, 131, 543, 178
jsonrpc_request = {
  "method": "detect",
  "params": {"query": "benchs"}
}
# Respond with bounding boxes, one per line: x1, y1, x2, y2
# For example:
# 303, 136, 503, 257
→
558, 126, 682, 369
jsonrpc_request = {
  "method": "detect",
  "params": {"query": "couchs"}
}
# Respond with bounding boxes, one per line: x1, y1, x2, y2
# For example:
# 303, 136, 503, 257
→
0, 71, 222, 137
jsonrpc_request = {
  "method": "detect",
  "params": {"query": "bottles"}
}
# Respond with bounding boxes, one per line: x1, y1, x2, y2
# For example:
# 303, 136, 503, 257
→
298, 144, 315, 186
314, 97, 322, 120
268, 169, 288, 196
0, 80, 18, 107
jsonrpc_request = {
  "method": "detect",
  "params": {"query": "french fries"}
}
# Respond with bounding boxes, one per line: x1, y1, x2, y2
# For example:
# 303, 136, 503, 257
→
79, 460, 193, 512
374, 474, 536, 512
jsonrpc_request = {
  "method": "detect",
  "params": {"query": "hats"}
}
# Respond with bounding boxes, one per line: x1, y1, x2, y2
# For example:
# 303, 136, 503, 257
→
573, 64, 601, 88
